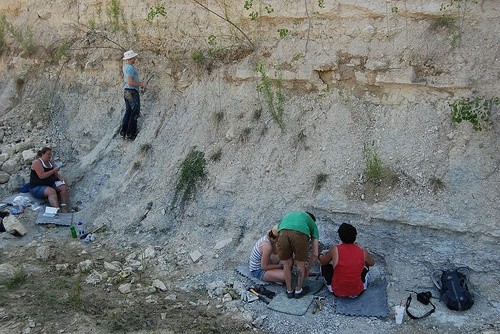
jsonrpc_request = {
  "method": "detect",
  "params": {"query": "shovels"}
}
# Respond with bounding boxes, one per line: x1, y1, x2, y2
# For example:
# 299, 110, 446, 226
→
144, 202, 153, 215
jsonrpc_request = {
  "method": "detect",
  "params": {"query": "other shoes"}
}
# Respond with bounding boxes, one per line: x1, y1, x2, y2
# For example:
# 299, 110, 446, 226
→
125, 134, 131, 139
61, 205, 68, 213
121, 132, 126, 138
57, 209, 61, 213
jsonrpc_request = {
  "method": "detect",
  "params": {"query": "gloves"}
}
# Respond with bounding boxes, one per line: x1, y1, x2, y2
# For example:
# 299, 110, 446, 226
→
318, 250, 329, 260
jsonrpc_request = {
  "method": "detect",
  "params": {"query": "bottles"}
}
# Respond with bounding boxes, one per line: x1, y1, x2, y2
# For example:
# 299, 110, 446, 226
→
77, 221, 85, 237
69, 225, 77, 238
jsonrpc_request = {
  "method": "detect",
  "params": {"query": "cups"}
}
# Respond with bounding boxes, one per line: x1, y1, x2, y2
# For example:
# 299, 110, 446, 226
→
395, 306, 405, 324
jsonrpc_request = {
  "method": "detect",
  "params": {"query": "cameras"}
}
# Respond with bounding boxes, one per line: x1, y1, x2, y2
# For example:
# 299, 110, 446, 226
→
417, 291, 431, 304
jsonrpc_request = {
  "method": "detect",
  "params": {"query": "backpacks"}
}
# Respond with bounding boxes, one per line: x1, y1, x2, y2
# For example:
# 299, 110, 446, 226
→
431, 267, 473, 311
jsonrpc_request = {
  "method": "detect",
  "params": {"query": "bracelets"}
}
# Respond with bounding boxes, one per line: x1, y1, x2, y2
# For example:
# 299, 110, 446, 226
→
318, 254, 324, 260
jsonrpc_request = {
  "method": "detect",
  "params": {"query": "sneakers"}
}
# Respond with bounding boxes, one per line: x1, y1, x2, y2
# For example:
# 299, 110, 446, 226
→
286, 289, 295, 298
294, 286, 310, 299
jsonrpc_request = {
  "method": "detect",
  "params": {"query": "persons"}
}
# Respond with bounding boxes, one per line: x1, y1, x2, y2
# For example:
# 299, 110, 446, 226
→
29, 147, 68, 207
120, 50, 144, 138
249, 224, 285, 281
277, 211, 319, 299
319, 223, 376, 298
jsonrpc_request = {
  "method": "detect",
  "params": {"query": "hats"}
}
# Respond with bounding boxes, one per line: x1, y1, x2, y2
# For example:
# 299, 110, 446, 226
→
122, 50, 138, 60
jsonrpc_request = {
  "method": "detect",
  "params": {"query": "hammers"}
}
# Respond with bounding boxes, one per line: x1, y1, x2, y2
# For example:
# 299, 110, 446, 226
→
312, 296, 325, 314
91, 222, 107, 233
